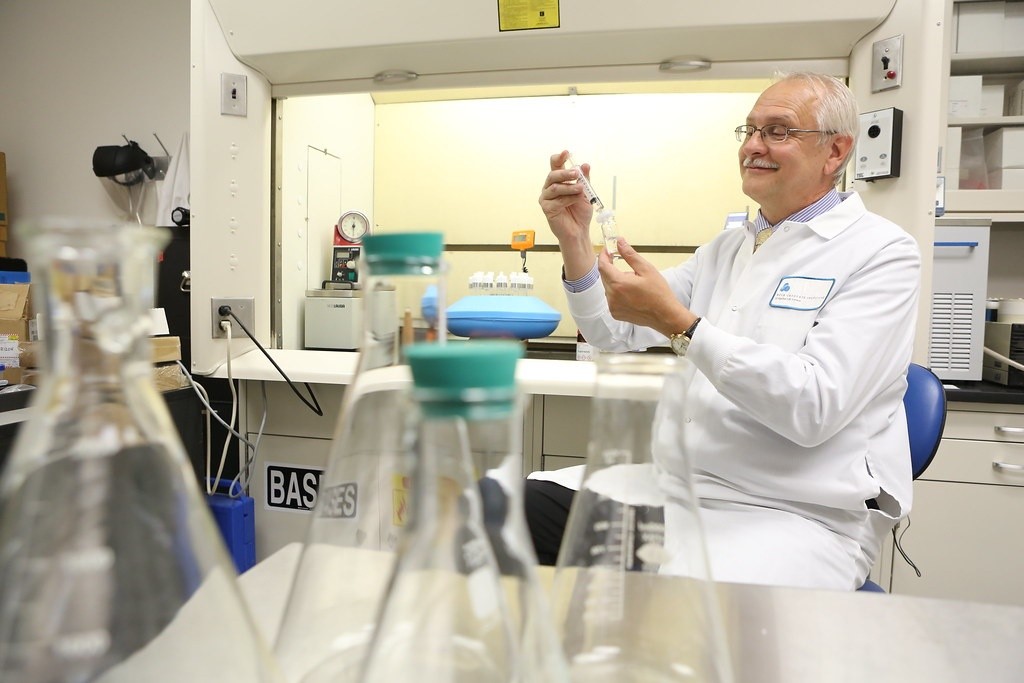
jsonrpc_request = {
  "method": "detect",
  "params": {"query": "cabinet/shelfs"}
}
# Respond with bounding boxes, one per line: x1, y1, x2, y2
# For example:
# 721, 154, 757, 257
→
935, 49, 1024, 225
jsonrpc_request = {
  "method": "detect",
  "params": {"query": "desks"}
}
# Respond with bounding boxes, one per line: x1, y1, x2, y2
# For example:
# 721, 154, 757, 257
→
96, 541, 1024, 683
203, 349, 668, 403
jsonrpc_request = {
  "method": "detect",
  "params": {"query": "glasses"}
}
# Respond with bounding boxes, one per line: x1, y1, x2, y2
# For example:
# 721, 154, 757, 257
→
735, 123, 837, 149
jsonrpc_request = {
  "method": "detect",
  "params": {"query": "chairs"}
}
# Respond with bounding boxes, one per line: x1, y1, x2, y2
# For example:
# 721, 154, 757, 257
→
853, 359, 947, 590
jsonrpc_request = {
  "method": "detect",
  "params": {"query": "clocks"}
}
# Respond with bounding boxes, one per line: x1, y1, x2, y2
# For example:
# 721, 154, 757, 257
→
337, 210, 371, 245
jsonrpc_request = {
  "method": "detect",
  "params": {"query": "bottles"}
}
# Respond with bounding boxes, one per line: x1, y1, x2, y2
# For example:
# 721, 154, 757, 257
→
0, 219, 735, 683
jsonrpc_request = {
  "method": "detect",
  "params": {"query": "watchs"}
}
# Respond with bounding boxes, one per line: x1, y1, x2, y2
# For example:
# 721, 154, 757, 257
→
671, 317, 701, 356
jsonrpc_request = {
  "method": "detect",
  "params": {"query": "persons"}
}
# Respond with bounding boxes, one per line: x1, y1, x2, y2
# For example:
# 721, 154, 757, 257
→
539, 72, 921, 591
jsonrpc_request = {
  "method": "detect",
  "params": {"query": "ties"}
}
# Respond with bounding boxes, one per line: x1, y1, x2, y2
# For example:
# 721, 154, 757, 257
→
753, 227, 773, 254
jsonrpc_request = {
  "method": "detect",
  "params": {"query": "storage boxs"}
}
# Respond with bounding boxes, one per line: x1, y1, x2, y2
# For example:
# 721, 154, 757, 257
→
943, 75, 1024, 190
0, 151, 183, 397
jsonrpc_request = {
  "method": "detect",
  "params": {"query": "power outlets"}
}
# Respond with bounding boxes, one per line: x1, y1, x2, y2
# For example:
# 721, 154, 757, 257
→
211, 296, 256, 340
